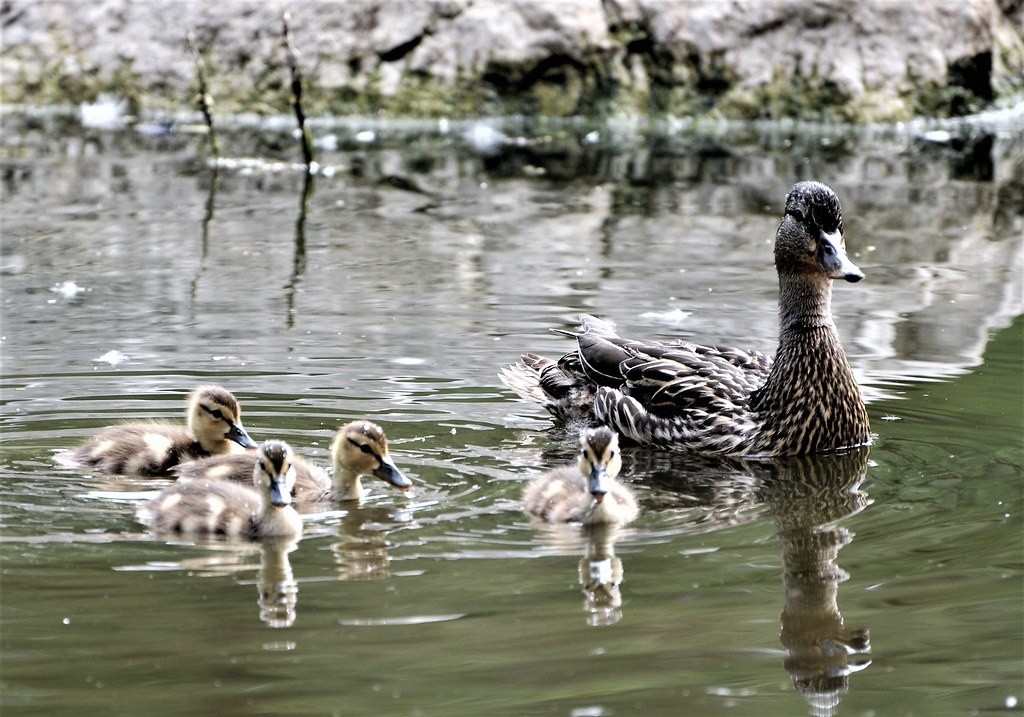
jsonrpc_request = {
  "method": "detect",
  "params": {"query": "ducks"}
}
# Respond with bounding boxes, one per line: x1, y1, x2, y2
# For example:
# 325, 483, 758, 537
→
54, 384, 412, 543
49, 524, 299, 627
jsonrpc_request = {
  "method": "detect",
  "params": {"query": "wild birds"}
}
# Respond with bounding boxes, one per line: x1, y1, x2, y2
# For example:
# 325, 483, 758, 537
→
497, 170, 872, 527
515, 429, 874, 710
